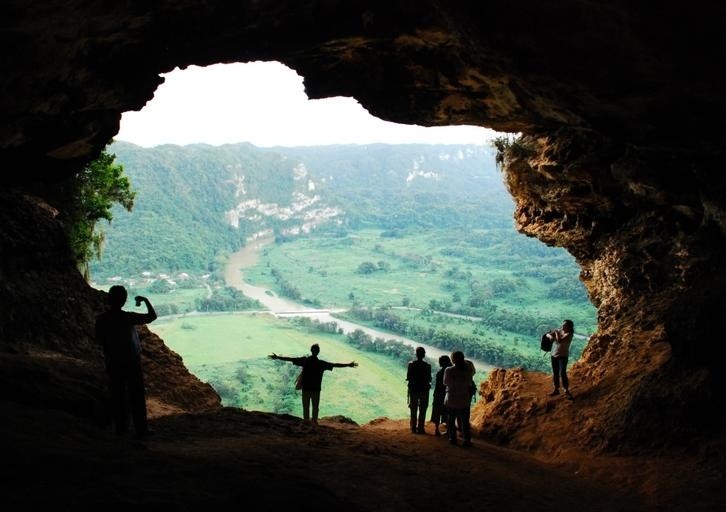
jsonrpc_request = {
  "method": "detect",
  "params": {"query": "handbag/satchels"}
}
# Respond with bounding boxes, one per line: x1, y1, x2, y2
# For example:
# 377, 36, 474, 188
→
540, 333, 555, 352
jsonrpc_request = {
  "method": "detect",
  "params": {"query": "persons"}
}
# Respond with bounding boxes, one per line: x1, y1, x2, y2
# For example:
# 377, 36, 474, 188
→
405, 346, 433, 434
267, 344, 359, 422
443, 351, 476, 446
92, 285, 158, 442
433, 355, 450, 436
550, 320, 574, 398
